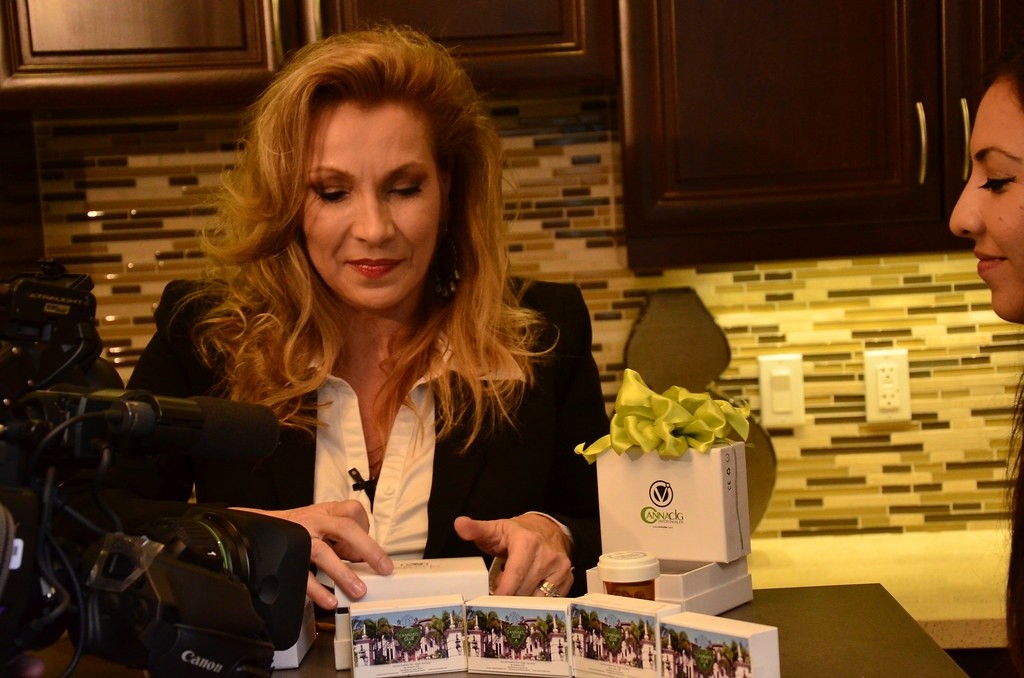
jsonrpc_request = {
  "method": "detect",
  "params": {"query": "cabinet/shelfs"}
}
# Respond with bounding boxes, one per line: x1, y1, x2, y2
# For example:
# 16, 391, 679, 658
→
0, 0, 1024, 272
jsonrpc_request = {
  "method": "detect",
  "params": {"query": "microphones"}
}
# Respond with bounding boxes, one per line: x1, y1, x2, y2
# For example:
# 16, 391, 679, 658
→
104, 397, 280, 464
348, 467, 365, 482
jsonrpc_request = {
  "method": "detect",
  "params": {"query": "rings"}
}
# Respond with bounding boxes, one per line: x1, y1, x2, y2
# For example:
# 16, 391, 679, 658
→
537, 578, 558, 599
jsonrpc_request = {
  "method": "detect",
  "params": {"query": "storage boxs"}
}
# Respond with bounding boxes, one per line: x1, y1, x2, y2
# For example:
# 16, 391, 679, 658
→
585, 557, 753, 617
348, 591, 781, 678
332, 555, 487, 668
272, 597, 316, 670
595, 441, 752, 564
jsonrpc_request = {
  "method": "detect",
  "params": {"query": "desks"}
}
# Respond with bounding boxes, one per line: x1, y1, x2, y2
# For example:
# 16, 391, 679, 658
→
31, 582, 969, 678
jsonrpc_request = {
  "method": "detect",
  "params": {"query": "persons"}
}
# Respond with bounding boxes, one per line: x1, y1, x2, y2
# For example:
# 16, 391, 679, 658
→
125, 19, 612, 617
950, 55, 1024, 678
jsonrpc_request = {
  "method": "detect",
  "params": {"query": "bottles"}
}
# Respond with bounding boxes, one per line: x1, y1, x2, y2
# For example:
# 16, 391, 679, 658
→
597, 550, 660, 600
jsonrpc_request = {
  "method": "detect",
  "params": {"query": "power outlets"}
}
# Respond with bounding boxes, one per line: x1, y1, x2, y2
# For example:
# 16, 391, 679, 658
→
863, 349, 912, 425
